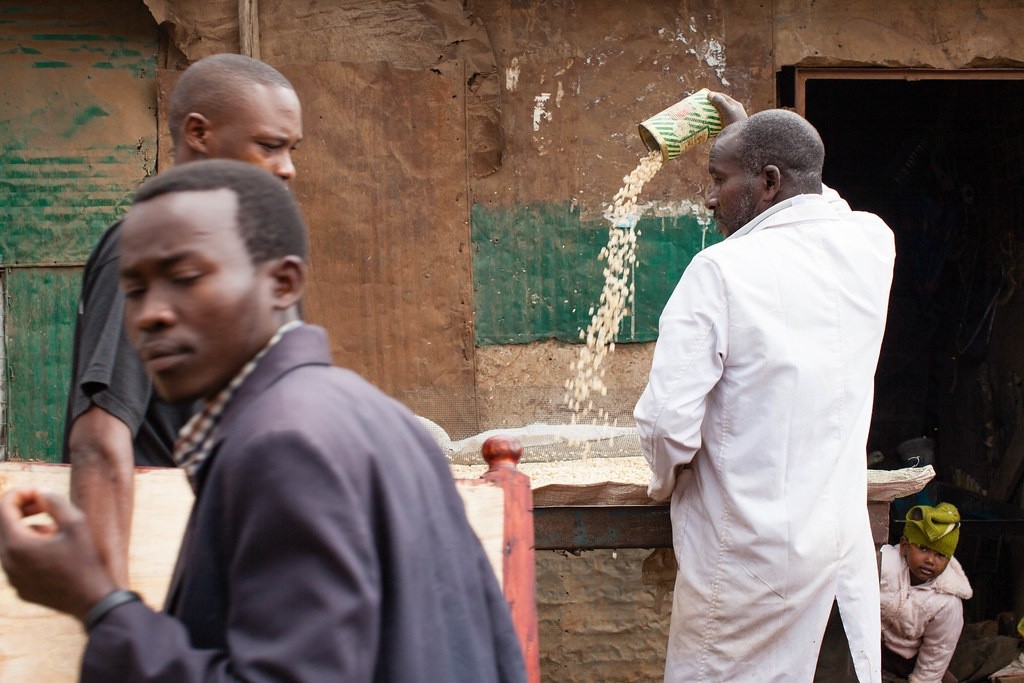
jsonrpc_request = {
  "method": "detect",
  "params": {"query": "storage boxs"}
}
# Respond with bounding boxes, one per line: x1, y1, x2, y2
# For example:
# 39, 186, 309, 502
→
889, 478, 1024, 623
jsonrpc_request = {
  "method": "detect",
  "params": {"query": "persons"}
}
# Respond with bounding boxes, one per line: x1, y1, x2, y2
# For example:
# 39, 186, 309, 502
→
636, 89, 895, 683
0, 156, 527, 682
60, 54, 304, 590
876, 501, 974, 683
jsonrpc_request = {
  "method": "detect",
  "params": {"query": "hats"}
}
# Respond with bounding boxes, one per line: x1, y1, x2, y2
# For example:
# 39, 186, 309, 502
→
903, 502, 961, 560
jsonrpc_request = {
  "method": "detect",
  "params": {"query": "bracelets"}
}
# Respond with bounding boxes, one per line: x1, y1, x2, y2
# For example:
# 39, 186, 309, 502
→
82, 588, 143, 635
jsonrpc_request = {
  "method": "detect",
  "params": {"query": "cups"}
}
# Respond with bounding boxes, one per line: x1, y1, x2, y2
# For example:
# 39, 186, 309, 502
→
638, 88, 721, 163
